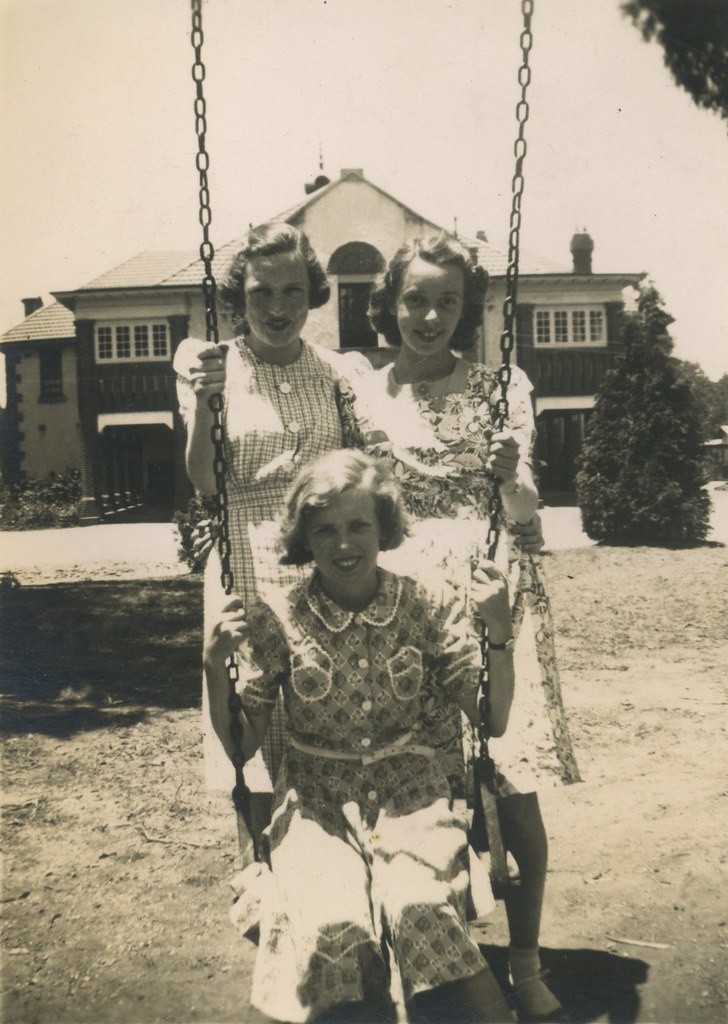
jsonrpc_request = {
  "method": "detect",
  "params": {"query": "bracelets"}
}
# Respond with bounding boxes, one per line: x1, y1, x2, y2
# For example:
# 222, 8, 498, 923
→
502, 481, 523, 497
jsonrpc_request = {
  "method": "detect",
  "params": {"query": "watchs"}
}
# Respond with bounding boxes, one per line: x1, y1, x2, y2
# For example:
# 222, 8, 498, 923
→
488, 636, 516, 651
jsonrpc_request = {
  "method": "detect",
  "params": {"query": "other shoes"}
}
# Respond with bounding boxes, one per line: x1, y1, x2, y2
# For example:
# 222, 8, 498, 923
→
505, 984, 570, 1024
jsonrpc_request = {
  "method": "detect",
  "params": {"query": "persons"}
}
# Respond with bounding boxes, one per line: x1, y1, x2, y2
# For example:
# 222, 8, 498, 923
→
173, 221, 377, 868
190, 233, 583, 1024
203, 451, 516, 1023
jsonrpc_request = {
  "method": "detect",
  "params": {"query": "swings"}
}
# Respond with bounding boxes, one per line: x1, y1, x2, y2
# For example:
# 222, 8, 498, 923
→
189, 0, 540, 942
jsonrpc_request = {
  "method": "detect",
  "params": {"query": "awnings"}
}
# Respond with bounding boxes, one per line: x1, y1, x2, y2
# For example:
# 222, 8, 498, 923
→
534, 397, 602, 414
